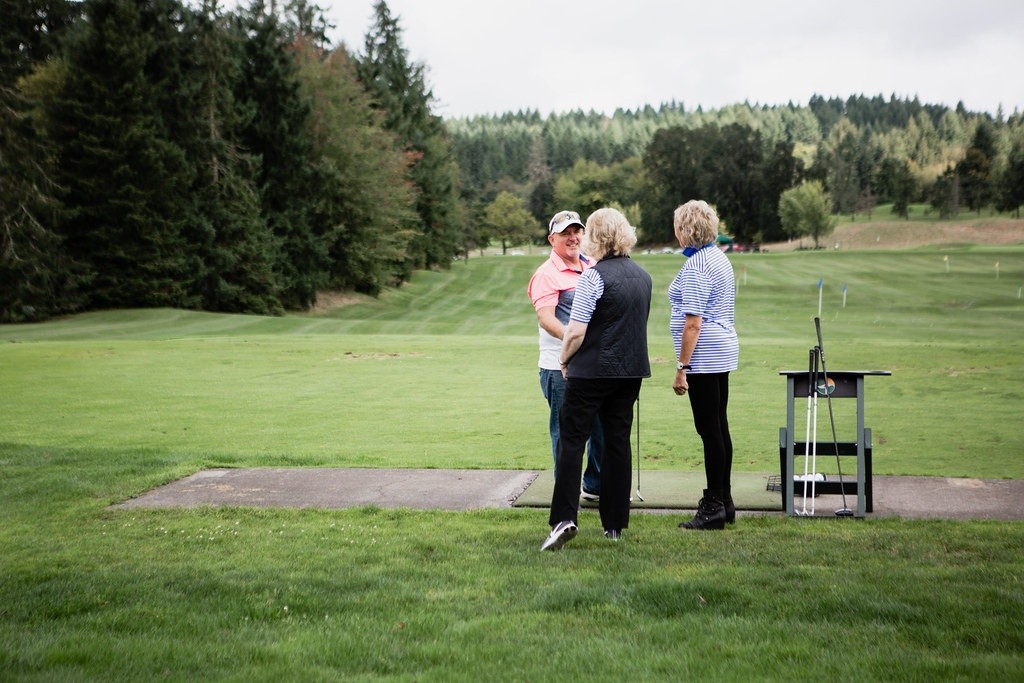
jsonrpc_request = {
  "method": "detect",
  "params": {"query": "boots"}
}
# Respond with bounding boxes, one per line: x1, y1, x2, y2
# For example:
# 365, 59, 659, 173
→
725, 497, 736, 523
678, 489, 725, 530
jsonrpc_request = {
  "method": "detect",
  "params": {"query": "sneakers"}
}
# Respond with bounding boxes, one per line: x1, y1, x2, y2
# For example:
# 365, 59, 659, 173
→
605, 530, 622, 542
541, 521, 578, 552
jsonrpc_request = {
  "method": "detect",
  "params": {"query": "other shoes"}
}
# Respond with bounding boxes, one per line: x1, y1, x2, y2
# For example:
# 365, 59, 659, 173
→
582, 486, 632, 502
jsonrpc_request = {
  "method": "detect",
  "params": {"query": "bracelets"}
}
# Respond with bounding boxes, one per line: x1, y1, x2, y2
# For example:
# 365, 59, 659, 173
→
559, 357, 567, 365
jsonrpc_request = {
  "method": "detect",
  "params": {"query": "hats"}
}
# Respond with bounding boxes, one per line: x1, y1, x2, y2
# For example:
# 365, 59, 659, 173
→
549, 211, 585, 235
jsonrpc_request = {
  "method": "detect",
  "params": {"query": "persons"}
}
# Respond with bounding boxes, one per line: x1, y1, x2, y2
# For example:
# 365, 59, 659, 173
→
528, 210, 635, 503
668, 199, 739, 530
541, 208, 652, 550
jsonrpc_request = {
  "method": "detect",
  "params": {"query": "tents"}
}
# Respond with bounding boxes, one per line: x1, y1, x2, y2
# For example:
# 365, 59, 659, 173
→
717, 234, 734, 250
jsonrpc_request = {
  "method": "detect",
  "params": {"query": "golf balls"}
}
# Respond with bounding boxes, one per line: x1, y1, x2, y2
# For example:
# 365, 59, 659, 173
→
791, 473, 826, 483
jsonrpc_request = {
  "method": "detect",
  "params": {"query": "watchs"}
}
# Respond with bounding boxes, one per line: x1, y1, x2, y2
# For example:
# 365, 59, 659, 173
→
677, 362, 693, 371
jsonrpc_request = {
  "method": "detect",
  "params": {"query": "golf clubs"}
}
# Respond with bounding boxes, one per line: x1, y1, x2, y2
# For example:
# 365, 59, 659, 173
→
814, 316, 854, 515
635, 396, 646, 504
803, 347, 820, 518
792, 348, 813, 515
